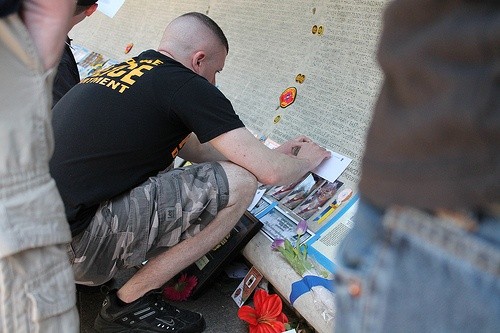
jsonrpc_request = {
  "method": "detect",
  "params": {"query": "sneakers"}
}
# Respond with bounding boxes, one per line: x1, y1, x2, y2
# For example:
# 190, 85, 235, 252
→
92, 287, 207, 333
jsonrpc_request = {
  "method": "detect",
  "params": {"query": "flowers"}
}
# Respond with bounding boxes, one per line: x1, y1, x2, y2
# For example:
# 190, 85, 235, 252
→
237, 288, 291, 333
164, 274, 198, 300
270, 220, 338, 313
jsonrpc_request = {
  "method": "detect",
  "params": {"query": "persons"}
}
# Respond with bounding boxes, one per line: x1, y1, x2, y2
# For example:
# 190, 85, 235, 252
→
336, 0, 500, 333
49, 12, 331, 333
0, 0, 77, 332
53, 0, 98, 108
